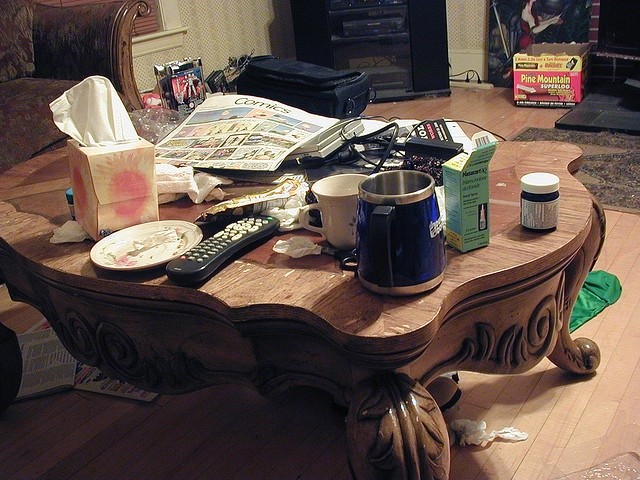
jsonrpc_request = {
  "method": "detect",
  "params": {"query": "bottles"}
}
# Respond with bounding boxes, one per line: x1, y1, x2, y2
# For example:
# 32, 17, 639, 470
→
65, 188, 75, 220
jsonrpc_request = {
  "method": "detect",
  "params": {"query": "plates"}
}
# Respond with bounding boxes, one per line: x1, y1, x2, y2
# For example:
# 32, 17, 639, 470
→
90, 220, 204, 272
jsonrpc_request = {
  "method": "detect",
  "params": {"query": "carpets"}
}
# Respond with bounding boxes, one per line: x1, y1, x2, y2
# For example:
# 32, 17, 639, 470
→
503, 127, 639, 216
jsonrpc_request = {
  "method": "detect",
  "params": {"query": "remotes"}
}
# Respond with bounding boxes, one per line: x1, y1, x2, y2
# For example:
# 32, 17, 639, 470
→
165, 215, 280, 285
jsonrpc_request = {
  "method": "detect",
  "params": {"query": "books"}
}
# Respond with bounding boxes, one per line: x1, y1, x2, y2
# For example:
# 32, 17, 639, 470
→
284, 123, 365, 161
290, 118, 361, 155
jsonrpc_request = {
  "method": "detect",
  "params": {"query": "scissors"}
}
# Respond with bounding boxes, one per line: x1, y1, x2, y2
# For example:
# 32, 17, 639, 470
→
315, 243, 359, 270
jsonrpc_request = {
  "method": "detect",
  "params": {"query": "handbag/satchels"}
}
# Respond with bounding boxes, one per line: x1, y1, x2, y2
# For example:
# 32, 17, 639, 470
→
236, 55, 376, 120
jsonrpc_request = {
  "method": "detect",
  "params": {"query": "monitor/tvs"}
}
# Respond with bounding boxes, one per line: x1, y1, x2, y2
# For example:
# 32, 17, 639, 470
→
597, 1, 639, 56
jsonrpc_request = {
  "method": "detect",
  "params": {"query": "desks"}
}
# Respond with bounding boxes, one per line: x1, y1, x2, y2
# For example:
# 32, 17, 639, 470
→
0, 105, 602, 478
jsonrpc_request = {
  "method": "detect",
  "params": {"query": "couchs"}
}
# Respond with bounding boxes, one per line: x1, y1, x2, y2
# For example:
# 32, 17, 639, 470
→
1, 0, 153, 180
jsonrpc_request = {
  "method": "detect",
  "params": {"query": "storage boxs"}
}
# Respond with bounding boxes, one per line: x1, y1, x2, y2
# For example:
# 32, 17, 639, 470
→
513, 42, 591, 109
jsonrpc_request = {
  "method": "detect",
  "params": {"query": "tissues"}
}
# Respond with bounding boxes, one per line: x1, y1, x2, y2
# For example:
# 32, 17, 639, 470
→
49, 75, 156, 240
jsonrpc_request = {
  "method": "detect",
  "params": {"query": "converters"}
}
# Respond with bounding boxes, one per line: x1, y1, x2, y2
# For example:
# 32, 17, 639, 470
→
406, 138, 463, 160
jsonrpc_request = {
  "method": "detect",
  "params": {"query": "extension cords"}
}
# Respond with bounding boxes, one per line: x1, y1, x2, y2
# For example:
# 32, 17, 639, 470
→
449, 79, 494, 91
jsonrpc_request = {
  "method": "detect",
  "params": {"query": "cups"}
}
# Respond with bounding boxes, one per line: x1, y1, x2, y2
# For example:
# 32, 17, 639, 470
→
358, 170, 446, 295
298, 173, 369, 252
